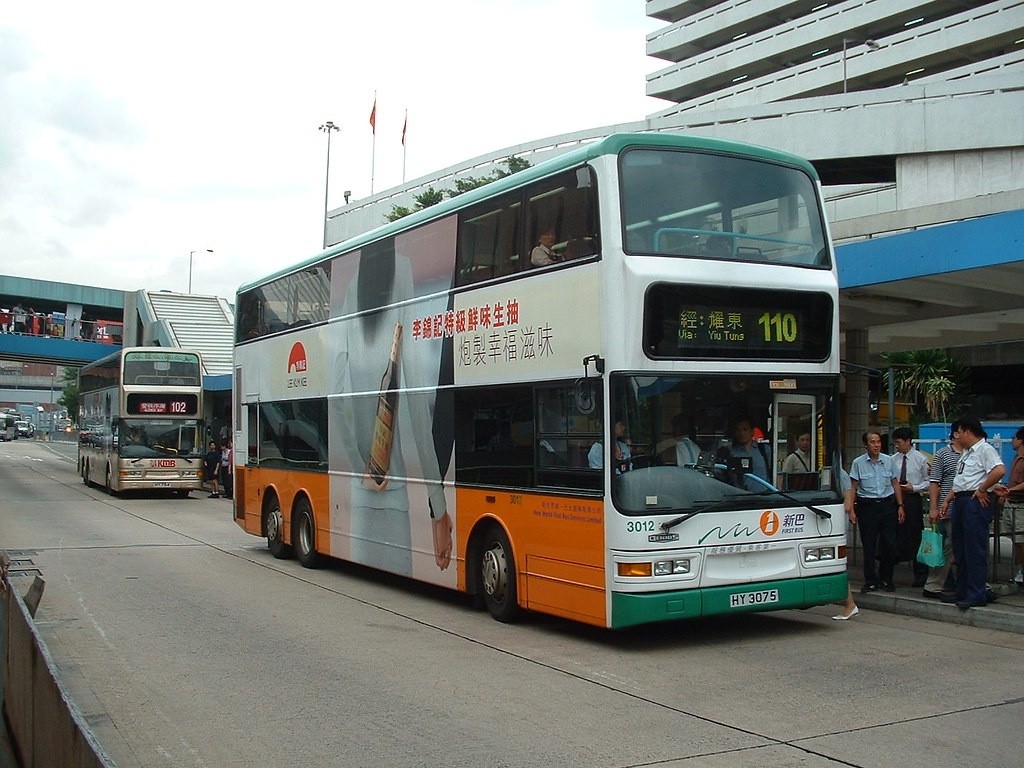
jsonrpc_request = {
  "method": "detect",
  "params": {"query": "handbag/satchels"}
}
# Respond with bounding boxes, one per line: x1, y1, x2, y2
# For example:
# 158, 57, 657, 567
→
916, 523, 946, 567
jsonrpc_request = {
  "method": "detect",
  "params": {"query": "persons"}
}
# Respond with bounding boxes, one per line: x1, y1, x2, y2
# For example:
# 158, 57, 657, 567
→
783, 428, 810, 472
848, 431, 906, 592
125, 428, 139, 441
1003, 427, 1024, 583
730, 414, 772, 482
531, 223, 565, 266
922, 418, 1010, 608
588, 413, 633, 475
206, 424, 233, 499
656, 412, 701, 466
329, 234, 455, 579
0, 303, 52, 337
832, 470, 859, 620
169, 370, 183, 384
875, 428, 931, 588
79, 311, 97, 340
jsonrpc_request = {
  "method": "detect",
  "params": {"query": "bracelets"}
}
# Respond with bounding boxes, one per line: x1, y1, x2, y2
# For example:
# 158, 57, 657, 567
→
978, 488, 987, 493
898, 504, 904, 509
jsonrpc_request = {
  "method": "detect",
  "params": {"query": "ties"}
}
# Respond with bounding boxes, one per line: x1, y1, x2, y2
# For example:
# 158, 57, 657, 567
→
899, 456, 908, 484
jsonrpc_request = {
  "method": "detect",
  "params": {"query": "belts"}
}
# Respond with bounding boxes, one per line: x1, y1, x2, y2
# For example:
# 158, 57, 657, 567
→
954, 490, 992, 497
858, 493, 896, 503
904, 492, 920, 496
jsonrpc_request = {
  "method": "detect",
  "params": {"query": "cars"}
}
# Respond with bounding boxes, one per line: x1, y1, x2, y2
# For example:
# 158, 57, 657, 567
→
0, 406, 79, 438
79, 424, 118, 447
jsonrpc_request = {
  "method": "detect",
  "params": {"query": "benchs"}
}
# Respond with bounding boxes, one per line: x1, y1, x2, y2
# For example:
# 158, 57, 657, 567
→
463, 229, 768, 284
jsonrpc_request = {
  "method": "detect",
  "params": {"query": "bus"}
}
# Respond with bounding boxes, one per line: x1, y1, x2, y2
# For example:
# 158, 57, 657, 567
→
0, 414, 15, 441
78, 347, 212, 497
229, 133, 883, 627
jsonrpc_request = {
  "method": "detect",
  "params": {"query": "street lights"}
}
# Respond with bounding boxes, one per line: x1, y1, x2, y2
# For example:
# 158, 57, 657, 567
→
189, 250, 213, 295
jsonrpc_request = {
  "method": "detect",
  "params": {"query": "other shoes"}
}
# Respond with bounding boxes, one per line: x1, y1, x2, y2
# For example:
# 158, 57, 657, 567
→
879, 577, 896, 592
955, 596, 986, 608
940, 592, 966, 603
1016, 570, 1024, 582
985, 588, 1000, 602
940, 580, 956, 591
911, 571, 928, 587
922, 589, 944, 598
207, 492, 233, 500
860, 579, 882, 592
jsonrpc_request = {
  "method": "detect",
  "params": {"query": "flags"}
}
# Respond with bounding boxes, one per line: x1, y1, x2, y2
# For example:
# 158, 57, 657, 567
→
402, 121, 406, 145
370, 101, 375, 133
